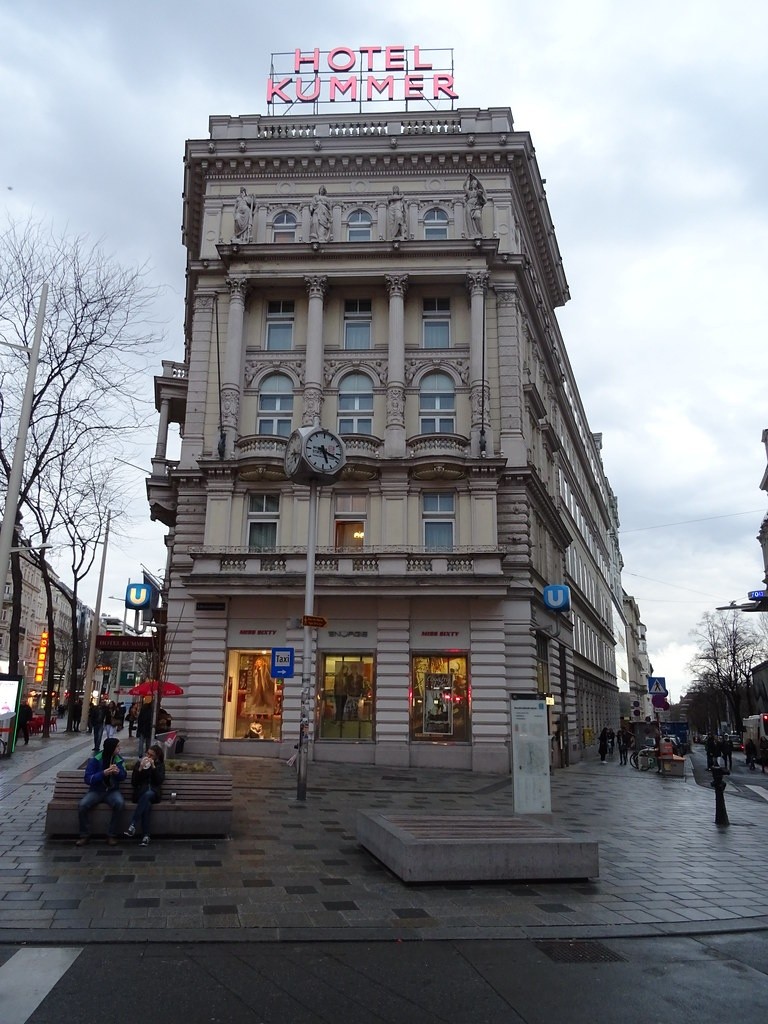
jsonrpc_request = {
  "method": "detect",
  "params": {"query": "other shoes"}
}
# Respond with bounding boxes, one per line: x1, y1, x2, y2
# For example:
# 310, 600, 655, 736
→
74, 729, 80, 732
139, 835, 150, 847
25, 741, 28, 745
106, 837, 117, 845
124, 824, 137, 836
601, 760, 608, 763
624, 761, 626, 765
75, 839, 89, 847
129, 735, 134, 738
92, 747, 99, 750
620, 762, 623, 765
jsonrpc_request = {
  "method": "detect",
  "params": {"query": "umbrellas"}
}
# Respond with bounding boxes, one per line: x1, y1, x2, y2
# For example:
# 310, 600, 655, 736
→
127, 681, 184, 696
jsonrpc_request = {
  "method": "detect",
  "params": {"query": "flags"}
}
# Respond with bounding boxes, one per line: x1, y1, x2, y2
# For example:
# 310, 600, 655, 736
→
141, 572, 160, 623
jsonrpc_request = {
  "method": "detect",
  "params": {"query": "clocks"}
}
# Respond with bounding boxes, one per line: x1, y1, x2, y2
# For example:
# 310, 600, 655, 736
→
304, 430, 343, 472
285, 433, 302, 474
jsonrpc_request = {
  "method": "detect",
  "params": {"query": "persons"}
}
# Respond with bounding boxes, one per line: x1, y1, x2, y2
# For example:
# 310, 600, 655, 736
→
15, 700, 33, 745
252, 658, 275, 707
745, 737, 758, 770
599, 727, 633, 765
463, 173, 487, 238
136, 699, 155, 758
387, 185, 408, 239
233, 187, 256, 244
59, 700, 135, 738
436, 693, 444, 714
76, 738, 128, 847
706, 733, 733, 770
309, 185, 334, 241
335, 664, 363, 721
91, 700, 106, 750
124, 745, 165, 847
760, 736, 768, 772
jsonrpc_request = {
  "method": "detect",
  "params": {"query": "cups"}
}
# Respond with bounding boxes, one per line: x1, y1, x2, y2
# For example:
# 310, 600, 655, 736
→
171, 793, 176, 804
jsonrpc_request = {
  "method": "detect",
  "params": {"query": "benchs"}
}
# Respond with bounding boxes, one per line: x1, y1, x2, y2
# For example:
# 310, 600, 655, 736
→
45, 769, 235, 836
